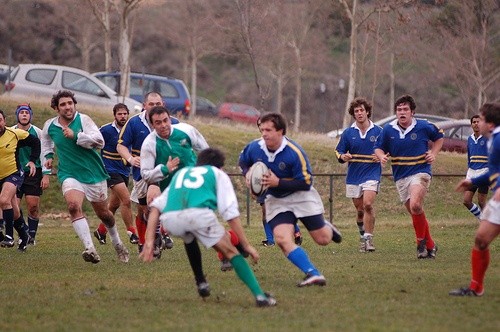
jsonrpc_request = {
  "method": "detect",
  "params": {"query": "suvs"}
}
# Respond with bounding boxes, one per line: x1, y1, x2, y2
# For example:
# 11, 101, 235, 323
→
66, 69, 190, 117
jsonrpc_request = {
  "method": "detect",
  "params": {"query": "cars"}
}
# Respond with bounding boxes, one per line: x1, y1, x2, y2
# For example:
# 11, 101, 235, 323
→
327, 112, 475, 155
191, 95, 218, 116
0, 63, 146, 121
217, 102, 262, 124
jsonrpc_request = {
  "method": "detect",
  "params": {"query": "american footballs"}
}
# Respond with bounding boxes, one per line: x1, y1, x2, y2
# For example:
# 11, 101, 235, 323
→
248, 162, 269, 197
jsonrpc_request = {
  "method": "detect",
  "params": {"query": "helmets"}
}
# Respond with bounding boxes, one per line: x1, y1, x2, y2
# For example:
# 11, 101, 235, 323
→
16, 104, 32, 123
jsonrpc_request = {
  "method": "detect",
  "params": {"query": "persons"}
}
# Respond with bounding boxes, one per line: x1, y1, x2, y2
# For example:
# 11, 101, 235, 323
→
374, 95, 445, 258
139, 147, 277, 307
461, 114, 488, 220
333, 95, 383, 254
254, 116, 306, 245
238, 113, 342, 286
38, 89, 130, 263
93, 91, 248, 270
0, 102, 52, 250
446, 102, 499, 295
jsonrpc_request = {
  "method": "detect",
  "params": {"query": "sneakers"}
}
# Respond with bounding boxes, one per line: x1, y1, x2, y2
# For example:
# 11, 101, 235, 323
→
359, 237, 369, 253
82, 248, 100, 263
449, 287, 484, 296
197, 275, 210, 296
236, 243, 249, 258
115, 243, 128, 263
263, 240, 275, 248
256, 291, 277, 307
324, 219, 341, 243
417, 239, 428, 258
299, 271, 326, 286
28, 237, 36, 246
17, 237, 22, 245
94, 227, 106, 244
18, 233, 30, 250
221, 261, 232, 271
365, 235, 375, 251
5, 234, 14, 246
294, 232, 303, 246
427, 244, 437, 258
127, 227, 173, 258
